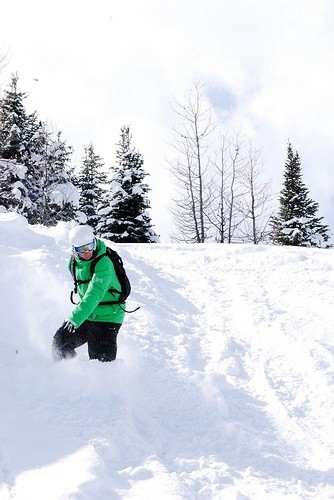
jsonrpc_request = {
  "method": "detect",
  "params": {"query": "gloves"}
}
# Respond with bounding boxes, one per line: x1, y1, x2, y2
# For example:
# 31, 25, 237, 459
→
59, 320, 75, 338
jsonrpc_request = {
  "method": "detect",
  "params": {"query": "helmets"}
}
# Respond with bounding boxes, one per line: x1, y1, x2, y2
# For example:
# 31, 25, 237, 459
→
69, 226, 95, 247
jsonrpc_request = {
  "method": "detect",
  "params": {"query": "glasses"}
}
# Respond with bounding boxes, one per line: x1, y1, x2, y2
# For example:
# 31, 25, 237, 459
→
74, 239, 95, 253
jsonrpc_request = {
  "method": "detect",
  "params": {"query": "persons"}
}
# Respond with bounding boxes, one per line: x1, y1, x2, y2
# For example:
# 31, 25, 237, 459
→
52, 227, 125, 362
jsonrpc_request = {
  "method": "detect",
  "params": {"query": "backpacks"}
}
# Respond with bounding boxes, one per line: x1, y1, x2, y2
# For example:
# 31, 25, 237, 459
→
70, 247, 131, 305
